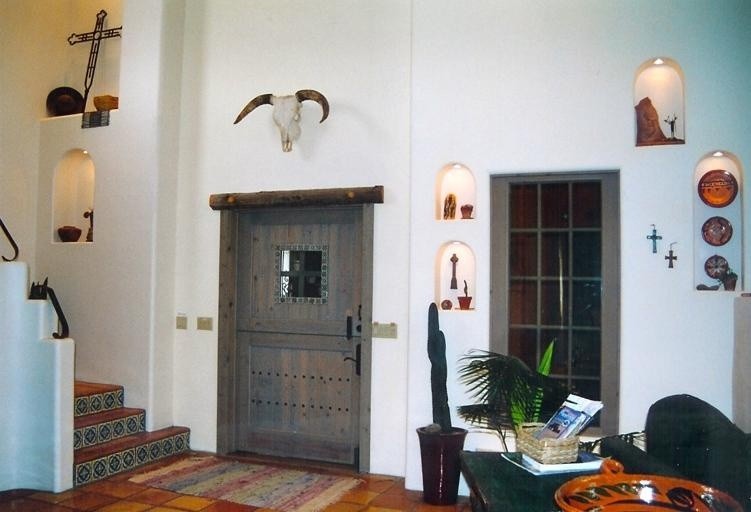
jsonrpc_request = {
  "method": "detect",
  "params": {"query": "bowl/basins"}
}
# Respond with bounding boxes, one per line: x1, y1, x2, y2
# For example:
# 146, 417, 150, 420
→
93, 96, 118, 111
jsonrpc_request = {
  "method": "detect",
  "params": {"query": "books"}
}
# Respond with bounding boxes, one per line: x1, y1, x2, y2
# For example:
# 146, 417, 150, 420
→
501, 451, 606, 478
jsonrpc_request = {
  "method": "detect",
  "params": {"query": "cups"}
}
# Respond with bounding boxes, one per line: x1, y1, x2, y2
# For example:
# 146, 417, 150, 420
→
461, 204, 472, 218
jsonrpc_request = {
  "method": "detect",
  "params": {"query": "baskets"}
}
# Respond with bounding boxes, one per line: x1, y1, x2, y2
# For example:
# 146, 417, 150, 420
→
516, 421, 579, 465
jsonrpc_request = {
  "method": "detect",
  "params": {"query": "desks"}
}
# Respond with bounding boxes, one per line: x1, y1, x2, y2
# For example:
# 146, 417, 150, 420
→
449, 450, 628, 512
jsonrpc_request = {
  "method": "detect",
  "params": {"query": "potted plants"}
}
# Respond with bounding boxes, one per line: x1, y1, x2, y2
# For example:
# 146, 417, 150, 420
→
458, 280, 472, 310
405, 303, 469, 504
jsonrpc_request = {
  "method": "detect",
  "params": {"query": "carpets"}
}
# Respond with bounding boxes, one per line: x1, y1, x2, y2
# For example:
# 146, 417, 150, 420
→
128, 456, 365, 512
